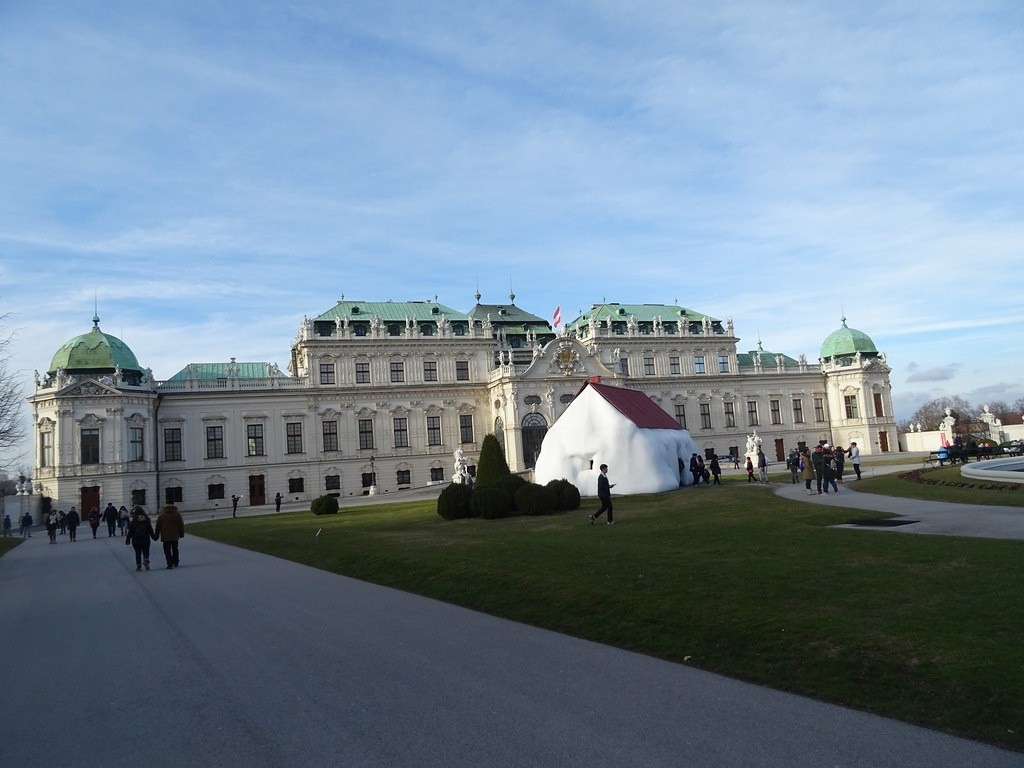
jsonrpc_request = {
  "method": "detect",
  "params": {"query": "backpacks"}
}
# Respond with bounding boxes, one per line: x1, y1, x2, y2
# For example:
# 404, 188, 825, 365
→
134, 523, 149, 544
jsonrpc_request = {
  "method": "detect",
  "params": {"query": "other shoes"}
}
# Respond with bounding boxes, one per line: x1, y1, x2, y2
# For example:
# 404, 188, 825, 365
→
135, 564, 142, 572
174, 563, 178, 567
49, 541, 57, 544
855, 477, 861, 481
165, 564, 173, 569
143, 560, 150, 570
836, 479, 845, 483
94, 536, 96, 539
606, 520, 615, 525
588, 514, 597, 525
819, 492, 830, 496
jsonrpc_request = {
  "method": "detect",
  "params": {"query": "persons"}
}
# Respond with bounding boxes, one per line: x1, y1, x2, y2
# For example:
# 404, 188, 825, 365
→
589, 464, 616, 525
34, 364, 152, 388
787, 440, 861, 495
88, 506, 103, 539
225, 362, 278, 377
4, 515, 12, 537
496, 328, 543, 365
155, 499, 185, 569
19, 512, 33, 537
125, 503, 155, 571
690, 453, 722, 488
938, 435, 1024, 466
275, 492, 283, 512
734, 449, 769, 483
303, 313, 494, 332
746, 431, 762, 452
102, 503, 118, 537
576, 316, 733, 335
46, 507, 80, 544
117, 506, 130, 536
751, 350, 886, 365
232, 495, 240, 517
455, 457, 468, 476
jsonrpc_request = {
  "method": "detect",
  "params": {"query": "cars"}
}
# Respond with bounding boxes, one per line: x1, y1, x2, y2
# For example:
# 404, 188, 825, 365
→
716, 455, 735, 463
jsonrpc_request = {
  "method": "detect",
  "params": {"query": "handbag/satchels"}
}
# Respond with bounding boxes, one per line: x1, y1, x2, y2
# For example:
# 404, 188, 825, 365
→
801, 460, 804, 472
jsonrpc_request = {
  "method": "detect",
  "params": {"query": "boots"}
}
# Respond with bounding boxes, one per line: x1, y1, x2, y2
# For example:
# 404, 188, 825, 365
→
806, 488, 816, 495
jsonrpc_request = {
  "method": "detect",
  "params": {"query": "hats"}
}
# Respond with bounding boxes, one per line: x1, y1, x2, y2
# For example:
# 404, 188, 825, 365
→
821, 444, 830, 449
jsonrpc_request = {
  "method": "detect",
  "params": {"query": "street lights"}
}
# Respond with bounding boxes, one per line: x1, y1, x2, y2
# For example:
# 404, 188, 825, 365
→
369, 455, 376, 486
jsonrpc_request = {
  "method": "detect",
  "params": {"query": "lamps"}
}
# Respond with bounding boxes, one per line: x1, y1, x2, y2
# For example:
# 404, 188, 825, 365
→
351, 306, 360, 315
431, 306, 440, 316
676, 310, 686, 317
498, 309, 507, 317
615, 308, 626, 316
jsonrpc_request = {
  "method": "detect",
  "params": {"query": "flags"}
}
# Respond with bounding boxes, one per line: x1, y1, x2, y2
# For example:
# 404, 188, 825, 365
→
553, 307, 560, 326
